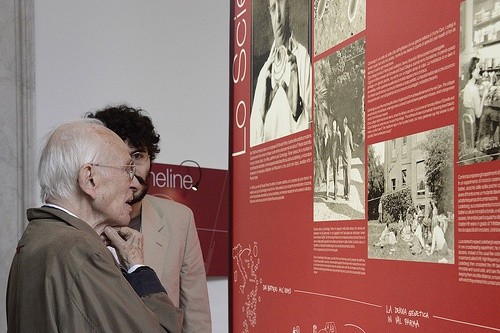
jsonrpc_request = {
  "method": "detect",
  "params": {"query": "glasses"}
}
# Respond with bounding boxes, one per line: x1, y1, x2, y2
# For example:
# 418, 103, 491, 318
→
131, 151, 152, 163
91, 160, 136, 180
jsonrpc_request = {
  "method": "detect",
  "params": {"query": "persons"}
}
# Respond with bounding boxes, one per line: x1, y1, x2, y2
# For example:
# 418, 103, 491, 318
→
87, 106, 212, 333
464, 57, 492, 147
6, 118, 184, 333
322, 116, 355, 200
373, 196, 449, 256
249, 0, 312, 147
